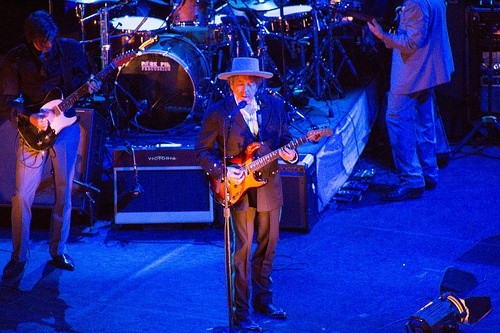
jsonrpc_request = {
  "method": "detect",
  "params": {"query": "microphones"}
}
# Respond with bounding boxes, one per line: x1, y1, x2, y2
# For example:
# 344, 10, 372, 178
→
133, 184, 142, 197
137, 100, 147, 117
226, 100, 248, 120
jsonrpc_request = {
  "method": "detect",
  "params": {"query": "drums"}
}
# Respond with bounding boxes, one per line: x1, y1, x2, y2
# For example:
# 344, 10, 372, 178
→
229, 1, 290, 31
171, 0, 214, 33
115, 33, 211, 133
111, 0, 168, 31
265, 5, 313, 34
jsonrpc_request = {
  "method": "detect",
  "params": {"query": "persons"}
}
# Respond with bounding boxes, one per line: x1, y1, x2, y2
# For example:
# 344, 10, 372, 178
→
0, 11, 102, 276
196, 57, 299, 317
367, 0, 455, 200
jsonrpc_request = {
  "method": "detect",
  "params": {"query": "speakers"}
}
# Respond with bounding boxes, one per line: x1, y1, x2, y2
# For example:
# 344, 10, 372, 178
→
275, 155, 315, 231
0, 107, 108, 229
112, 149, 219, 227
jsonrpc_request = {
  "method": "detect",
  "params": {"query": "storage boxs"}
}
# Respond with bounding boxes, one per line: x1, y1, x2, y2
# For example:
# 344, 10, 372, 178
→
113, 166, 214, 226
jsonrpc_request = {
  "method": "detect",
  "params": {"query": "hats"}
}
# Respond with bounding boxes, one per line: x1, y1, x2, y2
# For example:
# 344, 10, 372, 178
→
218, 57, 273, 79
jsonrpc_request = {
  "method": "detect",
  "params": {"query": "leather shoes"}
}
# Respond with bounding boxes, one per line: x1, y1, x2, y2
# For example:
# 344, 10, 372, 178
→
50, 254, 76, 271
3, 260, 26, 280
237, 312, 262, 332
253, 303, 287, 320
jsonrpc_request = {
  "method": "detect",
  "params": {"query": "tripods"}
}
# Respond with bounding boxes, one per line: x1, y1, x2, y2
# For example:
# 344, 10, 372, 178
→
452, 0, 500, 156
287, 2, 363, 119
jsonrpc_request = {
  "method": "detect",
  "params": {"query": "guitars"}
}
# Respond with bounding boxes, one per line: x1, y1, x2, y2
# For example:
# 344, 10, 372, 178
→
325, 3, 402, 36
210, 125, 334, 206
16, 48, 137, 152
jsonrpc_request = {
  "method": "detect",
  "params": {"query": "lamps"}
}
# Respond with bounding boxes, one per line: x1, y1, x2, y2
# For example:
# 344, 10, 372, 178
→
405, 267, 493, 333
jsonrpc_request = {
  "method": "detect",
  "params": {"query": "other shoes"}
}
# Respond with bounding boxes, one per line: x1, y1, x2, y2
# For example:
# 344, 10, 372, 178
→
385, 187, 423, 202
424, 178, 436, 190
